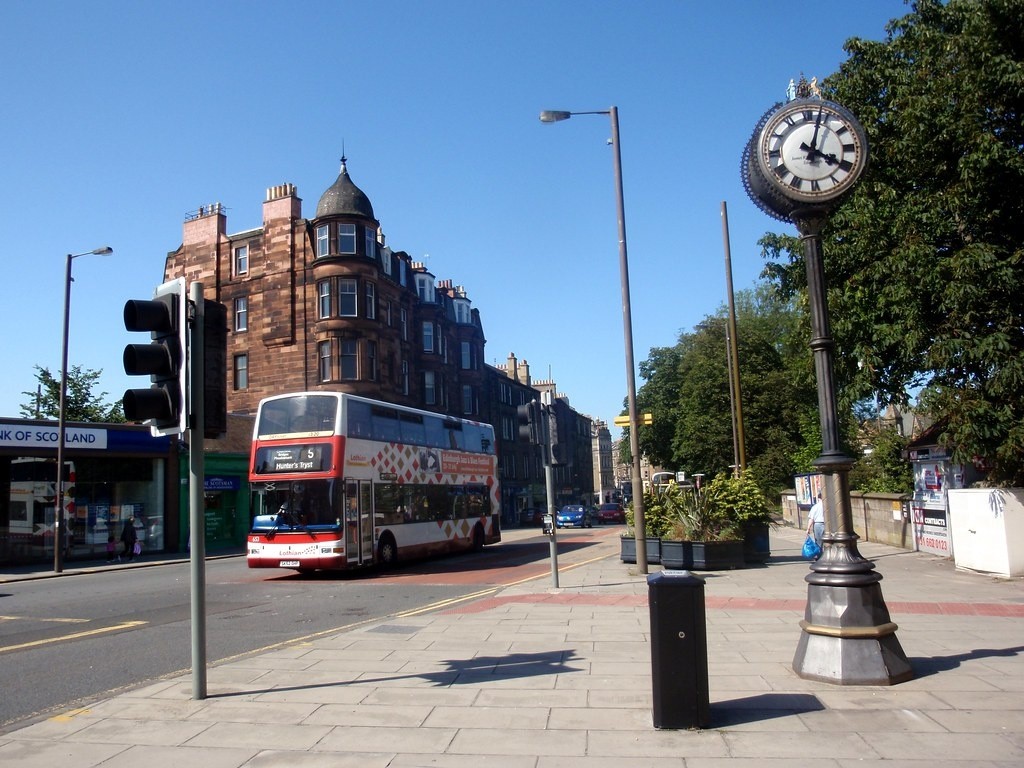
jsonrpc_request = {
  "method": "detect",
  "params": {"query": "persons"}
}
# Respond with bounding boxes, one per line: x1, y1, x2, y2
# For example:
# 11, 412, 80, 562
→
534, 511, 541, 527
119, 517, 138, 563
807, 493, 826, 561
105, 536, 119, 563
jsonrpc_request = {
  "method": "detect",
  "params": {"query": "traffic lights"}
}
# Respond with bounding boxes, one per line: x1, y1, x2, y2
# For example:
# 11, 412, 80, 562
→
122, 293, 181, 428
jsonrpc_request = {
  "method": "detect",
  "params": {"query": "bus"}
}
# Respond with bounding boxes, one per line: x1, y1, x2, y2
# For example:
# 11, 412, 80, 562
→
244, 391, 501, 575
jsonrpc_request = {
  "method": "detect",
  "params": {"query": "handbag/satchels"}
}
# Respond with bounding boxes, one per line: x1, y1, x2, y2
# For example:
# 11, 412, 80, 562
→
802, 535, 821, 561
134, 543, 141, 554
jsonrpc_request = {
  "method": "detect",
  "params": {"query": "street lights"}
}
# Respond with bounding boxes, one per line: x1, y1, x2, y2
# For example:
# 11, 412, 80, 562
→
52, 246, 113, 574
537, 106, 648, 573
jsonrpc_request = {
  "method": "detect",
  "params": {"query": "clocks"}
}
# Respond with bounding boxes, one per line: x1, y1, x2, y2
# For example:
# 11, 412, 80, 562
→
739, 70, 867, 225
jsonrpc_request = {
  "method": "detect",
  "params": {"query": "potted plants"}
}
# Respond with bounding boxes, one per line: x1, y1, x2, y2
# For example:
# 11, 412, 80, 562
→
657, 478, 746, 570
712, 466, 782, 563
620, 480, 684, 563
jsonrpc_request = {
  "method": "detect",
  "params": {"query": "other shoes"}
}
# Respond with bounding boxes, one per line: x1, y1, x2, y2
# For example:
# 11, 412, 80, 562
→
128, 560, 134, 562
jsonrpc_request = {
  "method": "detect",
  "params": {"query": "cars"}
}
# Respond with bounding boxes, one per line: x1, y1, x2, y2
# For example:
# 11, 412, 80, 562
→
556, 504, 593, 528
596, 503, 626, 524
519, 506, 561, 525
